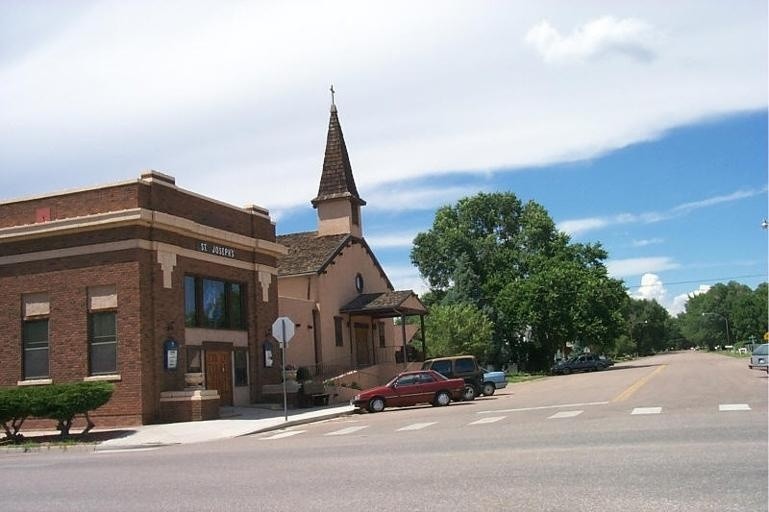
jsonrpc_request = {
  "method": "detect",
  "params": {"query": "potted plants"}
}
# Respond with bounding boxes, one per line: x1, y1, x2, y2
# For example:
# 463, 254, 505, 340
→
281, 364, 299, 381
183, 367, 207, 391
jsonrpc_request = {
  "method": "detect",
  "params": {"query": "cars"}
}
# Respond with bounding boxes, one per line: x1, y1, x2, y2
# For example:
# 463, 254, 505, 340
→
351, 355, 506, 412
551, 352, 608, 375
749, 344, 769, 374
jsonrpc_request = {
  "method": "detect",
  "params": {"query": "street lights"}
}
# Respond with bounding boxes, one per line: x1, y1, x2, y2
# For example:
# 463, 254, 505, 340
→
702, 312, 730, 344
630, 320, 648, 351
